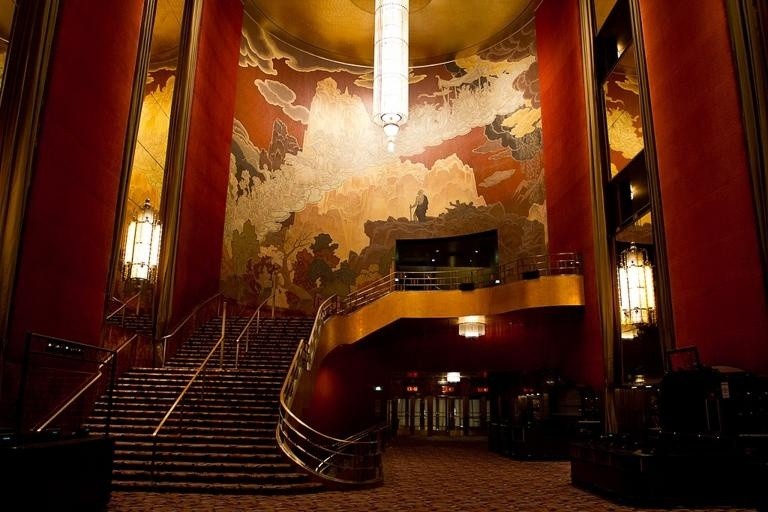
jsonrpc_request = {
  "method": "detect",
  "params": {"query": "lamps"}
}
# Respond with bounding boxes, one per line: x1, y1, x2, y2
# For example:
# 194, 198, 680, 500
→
119, 197, 163, 295
46, 339, 83, 356
491, 279, 500, 286
617, 241, 660, 340
456, 322, 487, 339
370, 0, 410, 156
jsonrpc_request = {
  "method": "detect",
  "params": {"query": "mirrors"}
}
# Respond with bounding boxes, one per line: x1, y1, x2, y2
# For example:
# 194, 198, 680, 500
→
99, 0, 202, 331
605, 209, 676, 389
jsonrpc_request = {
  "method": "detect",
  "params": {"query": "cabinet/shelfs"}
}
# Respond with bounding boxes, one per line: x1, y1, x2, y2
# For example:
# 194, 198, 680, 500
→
566, 443, 651, 508
0, 333, 118, 512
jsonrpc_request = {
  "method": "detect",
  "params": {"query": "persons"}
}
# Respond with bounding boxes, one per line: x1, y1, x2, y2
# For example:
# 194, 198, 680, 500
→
409, 190, 431, 220
